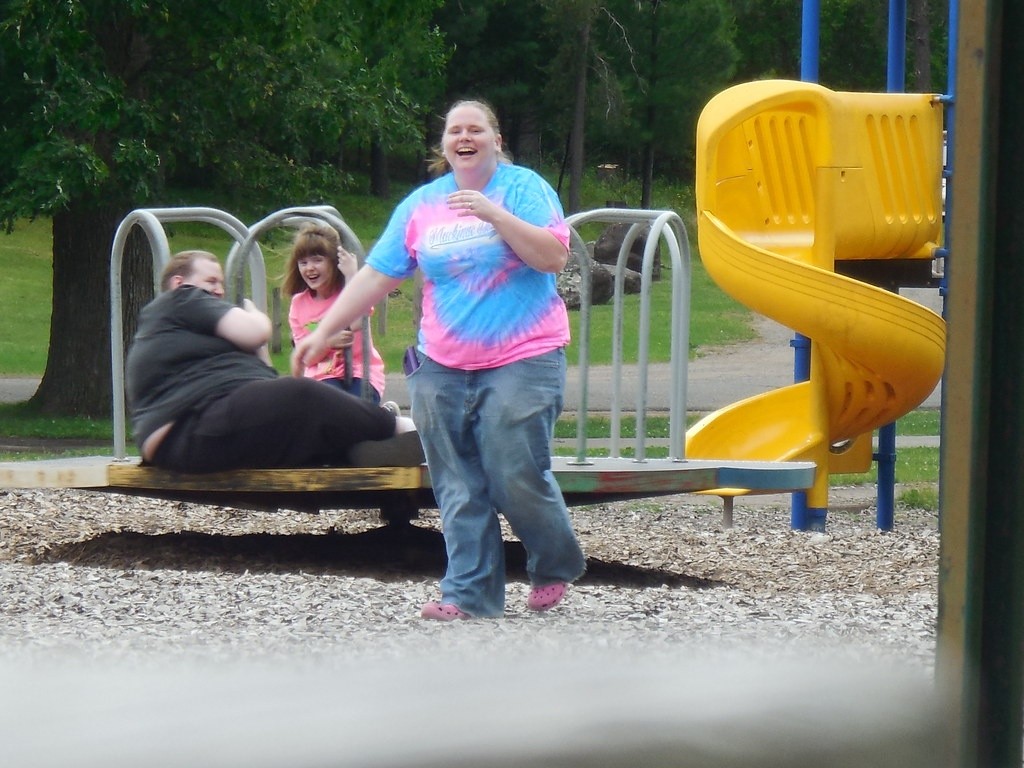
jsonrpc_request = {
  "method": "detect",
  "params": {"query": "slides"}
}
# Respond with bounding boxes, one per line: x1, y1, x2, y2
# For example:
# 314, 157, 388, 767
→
686, 78, 947, 498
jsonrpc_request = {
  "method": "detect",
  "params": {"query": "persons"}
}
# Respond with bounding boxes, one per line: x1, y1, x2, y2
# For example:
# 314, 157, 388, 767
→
124, 249, 425, 475
278, 222, 384, 405
289, 98, 588, 622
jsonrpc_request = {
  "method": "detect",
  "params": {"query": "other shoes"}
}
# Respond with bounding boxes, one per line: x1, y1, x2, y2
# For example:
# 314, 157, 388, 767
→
527, 579, 568, 610
381, 401, 402, 418
421, 602, 470, 620
349, 429, 428, 468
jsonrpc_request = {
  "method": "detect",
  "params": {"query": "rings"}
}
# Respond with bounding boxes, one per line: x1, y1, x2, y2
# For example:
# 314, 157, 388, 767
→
468, 202, 471, 209
